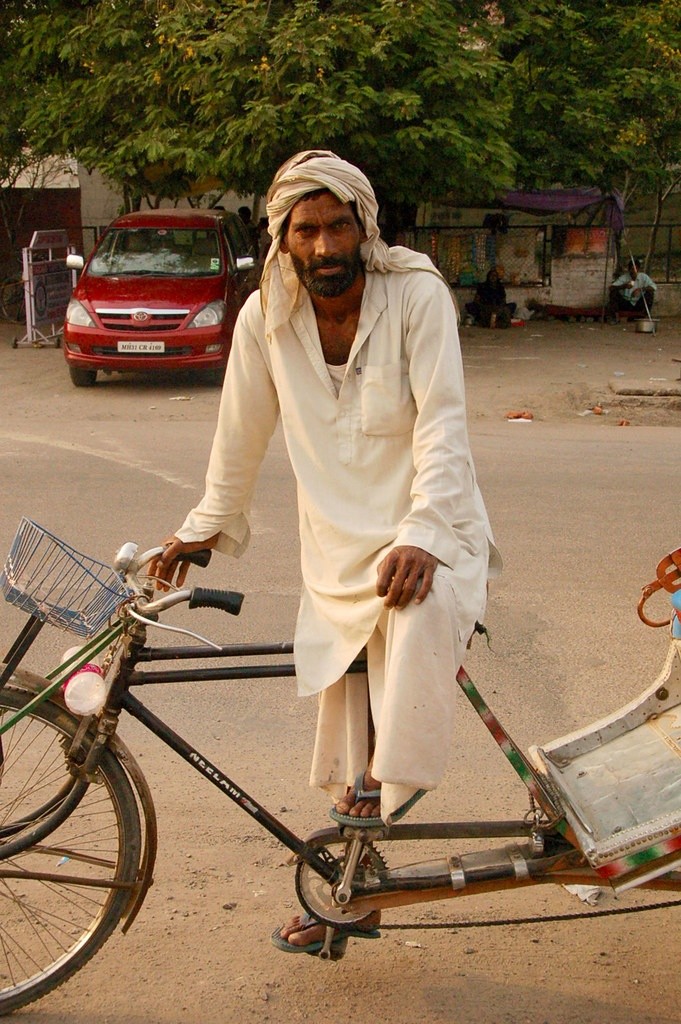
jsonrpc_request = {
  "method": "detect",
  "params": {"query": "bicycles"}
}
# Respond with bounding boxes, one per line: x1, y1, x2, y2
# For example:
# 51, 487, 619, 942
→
0, 249, 48, 326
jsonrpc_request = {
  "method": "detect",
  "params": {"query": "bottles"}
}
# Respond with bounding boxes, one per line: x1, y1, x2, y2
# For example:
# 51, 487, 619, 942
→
60, 645, 108, 716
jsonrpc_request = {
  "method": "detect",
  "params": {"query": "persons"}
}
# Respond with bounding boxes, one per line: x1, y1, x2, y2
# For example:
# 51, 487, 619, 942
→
144, 150, 497, 954
464, 264, 517, 330
602, 259, 656, 325
214, 206, 267, 266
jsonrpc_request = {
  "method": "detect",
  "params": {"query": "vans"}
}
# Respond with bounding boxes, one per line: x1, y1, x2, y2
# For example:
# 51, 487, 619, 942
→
60, 204, 262, 388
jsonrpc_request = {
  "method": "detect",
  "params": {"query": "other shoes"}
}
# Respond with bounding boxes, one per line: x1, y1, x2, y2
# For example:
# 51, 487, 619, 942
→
610, 318, 620, 325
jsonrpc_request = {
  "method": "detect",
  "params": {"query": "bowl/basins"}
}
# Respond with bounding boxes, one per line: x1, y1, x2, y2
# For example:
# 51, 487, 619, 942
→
634, 318, 660, 332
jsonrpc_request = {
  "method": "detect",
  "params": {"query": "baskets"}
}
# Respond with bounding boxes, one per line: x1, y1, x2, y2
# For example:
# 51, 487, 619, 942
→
0, 514, 129, 638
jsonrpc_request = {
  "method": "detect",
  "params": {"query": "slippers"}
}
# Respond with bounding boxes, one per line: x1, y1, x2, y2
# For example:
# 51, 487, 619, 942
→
329, 768, 428, 827
269, 912, 381, 952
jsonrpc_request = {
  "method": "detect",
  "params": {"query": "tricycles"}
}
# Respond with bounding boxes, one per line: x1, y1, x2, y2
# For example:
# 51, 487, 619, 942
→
0, 514, 681, 1016
